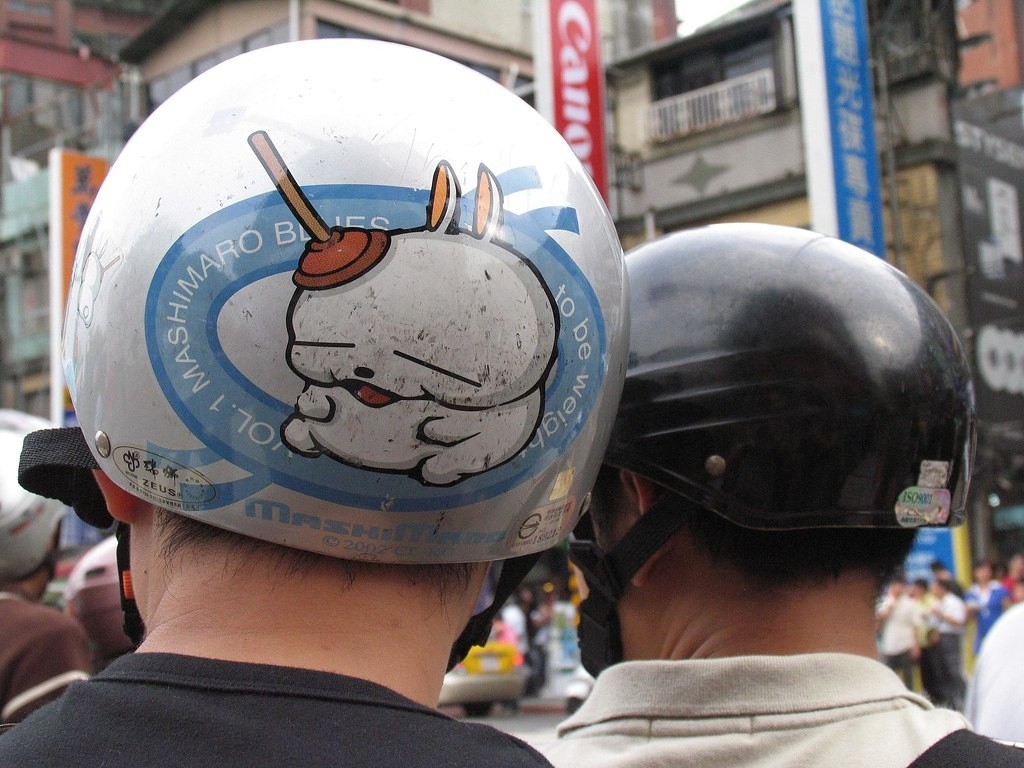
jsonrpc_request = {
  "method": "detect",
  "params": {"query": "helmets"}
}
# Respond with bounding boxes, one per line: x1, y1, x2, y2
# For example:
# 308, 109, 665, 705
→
601, 221, 977, 531
0, 406, 65, 582
61, 40, 632, 564
63, 532, 134, 660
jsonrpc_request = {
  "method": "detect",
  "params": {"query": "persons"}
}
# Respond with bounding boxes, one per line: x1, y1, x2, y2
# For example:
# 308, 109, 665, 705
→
873, 554, 1024, 745
0, 408, 144, 736
0, 37, 628, 768
502, 588, 574, 698
531, 221, 1024, 768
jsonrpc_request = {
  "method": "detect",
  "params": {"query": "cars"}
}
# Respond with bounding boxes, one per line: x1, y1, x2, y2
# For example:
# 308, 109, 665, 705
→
439, 624, 530, 720
